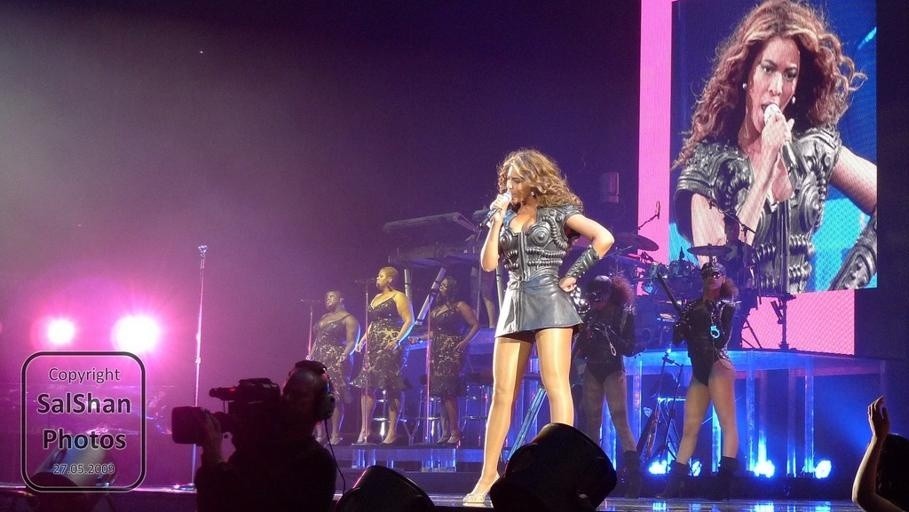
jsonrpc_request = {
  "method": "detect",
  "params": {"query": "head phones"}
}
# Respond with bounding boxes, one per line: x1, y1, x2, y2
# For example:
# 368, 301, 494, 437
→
288, 359, 336, 419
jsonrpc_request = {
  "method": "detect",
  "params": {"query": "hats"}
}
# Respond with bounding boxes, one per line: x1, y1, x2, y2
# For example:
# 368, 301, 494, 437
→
702, 262, 726, 272
588, 275, 612, 288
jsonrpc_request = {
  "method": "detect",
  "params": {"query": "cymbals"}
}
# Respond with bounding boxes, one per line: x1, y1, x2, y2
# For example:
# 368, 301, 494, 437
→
687, 246, 730, 257
615, 232, 658, 252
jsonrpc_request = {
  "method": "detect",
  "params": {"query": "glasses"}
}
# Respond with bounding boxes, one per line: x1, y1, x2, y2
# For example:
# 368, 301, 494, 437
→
703, 270, 721, 279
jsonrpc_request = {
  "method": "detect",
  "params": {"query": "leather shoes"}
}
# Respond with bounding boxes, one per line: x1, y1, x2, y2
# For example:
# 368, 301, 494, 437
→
462, 472, 501, 504
616, 451, 642, 498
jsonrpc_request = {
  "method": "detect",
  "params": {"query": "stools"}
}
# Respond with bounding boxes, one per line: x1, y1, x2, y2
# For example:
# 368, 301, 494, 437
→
371, 373, 507, 447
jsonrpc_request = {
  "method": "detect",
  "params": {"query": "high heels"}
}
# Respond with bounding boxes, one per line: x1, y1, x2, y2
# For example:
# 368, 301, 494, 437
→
705, 469, 738, 501
436, 432, 464, 449
655, 462, 689, 499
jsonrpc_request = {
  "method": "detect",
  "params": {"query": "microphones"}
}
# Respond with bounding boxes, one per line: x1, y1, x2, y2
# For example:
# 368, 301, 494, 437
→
764, 104, 801, 182
418, 286, 439, 292
481, 192, 513, 232
300, 298, 323, 306
354, 278, 377, 284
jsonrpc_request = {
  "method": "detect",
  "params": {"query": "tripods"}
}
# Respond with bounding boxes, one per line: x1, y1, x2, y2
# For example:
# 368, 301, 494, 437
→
642, 363, 685, 465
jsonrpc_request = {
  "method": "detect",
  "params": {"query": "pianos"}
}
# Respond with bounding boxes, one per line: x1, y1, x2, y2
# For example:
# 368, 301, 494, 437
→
384, 212, 482, 238
388, 247, 480, 268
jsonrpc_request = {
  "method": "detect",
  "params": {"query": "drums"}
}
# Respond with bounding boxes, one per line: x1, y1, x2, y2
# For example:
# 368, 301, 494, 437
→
667, 261, 697, 292
642, 264, 670, 299
634, 312, 676, 350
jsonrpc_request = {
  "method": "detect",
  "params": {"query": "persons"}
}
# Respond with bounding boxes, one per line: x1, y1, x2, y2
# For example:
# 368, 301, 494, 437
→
658, 264, 740, 501
672, 0, 878, 289
852, 395, 903, 512
408, 276, 480, 445
194, 361, 338, 511
462, 150, 615, 503
308, 290, 361, 445
353, 267, 415, 446
580, 275, 642, 499
